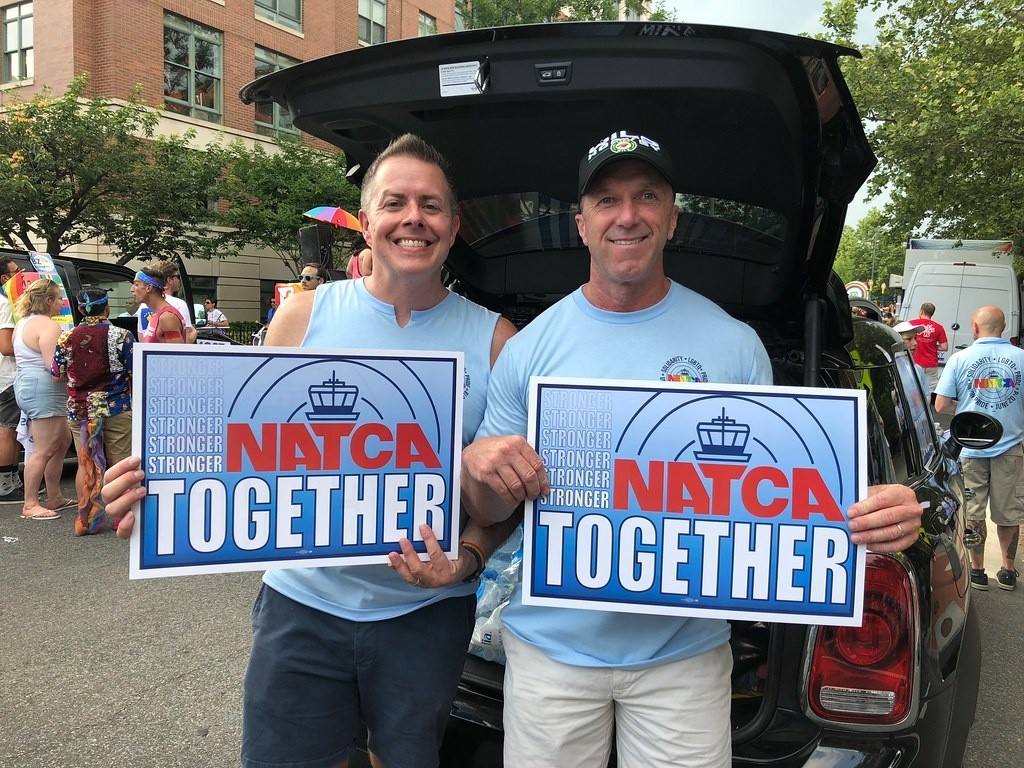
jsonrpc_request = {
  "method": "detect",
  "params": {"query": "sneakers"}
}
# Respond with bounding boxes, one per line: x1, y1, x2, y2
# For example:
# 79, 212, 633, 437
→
0, 488, 41, 504
970, 562, 989, 590
19, 484, 46, 494
995, 566, 1020, 590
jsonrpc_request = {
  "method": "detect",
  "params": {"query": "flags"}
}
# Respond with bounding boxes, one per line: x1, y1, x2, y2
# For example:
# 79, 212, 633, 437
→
0, 270, 26, 324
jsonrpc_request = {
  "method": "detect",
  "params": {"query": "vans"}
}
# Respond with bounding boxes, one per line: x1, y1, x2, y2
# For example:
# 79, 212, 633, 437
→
897, 262, 1021, 377
0, 248, 207, 462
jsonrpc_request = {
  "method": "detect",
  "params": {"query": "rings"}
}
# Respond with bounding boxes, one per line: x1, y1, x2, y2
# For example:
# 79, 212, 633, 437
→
413, 579, 420, 586
896, 524, 904, 537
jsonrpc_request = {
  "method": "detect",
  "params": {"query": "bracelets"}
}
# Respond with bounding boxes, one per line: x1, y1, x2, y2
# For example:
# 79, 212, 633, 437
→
461, 541, 486, 582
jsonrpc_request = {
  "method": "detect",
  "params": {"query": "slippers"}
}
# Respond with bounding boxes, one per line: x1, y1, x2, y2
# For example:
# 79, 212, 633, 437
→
54, 500, 79, 511
20, 510, 61, 520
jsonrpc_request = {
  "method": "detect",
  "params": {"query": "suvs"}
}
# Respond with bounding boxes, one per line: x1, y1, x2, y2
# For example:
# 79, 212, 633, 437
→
238, 21, 1006, 768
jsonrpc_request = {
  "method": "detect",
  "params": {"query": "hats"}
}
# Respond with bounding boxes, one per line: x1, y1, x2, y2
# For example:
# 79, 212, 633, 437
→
893, 321, 925, 333
578, 130, 676, 208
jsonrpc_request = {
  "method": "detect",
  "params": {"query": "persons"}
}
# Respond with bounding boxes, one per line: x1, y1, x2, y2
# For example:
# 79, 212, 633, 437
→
11, 280, 78, 519
460, 121, 922, 768
298, 262, 326, 291
204, 297, 229, 335
130, 267, 186, 343
908, 302, 948, 392
884, 318, 931, 412
101, 133, 523, 768
935, 305, 1024, 590
267, 296, 276, 325
51, 289, 138, 536
0, 256, 46, 505
137, 261, 197, 344
117, 296, 140, 317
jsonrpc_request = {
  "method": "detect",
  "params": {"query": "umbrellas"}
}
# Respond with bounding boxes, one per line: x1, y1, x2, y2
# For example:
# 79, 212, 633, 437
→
303, 206, 364, 247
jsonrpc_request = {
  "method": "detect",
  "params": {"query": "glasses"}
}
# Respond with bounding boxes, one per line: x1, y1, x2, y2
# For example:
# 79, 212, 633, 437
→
166, 275, 180, 281
0, 268, 20, 279
298, 275, 321, 281
205, 302, 209, 304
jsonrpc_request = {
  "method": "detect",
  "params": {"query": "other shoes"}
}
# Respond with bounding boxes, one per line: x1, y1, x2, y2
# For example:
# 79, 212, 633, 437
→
112, 508, 131, 530
